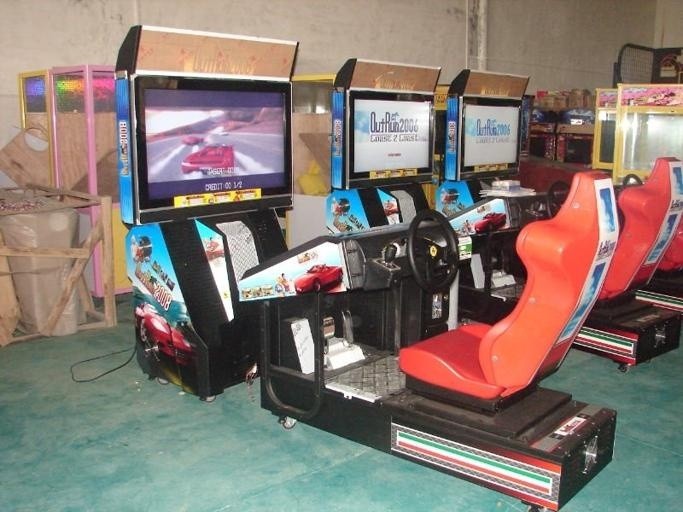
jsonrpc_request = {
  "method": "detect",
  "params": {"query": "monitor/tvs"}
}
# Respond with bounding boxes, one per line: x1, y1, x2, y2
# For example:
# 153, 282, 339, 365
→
530, 137, 545, 158
599, 114, 616, 163
460, 96, 522, 172
350, 90, 433, 179
624, 113, 683, 170
564, 139, 593, 164
135, 75, 292, 210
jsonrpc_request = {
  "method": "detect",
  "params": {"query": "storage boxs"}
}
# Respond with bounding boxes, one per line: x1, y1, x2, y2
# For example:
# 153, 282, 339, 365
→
537, 93, 594, 112
